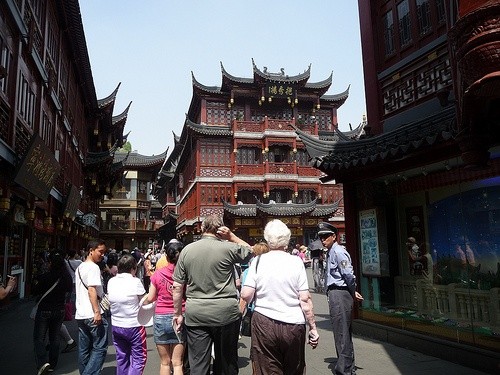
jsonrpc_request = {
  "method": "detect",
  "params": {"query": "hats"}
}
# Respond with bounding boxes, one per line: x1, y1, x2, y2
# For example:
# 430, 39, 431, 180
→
169, 239, 179, 243
137, 294, 156, 325
300, 245, 307, 253
317, 222, 337, 237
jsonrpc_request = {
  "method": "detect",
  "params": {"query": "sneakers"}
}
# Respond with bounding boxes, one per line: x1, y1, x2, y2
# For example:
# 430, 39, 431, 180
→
47, 367, 54, 372
37, 363, 50, 375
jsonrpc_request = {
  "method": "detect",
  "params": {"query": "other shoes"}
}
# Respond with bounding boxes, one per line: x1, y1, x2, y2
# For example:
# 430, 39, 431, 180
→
61, 340, 77, 353
46, 344, 50, 350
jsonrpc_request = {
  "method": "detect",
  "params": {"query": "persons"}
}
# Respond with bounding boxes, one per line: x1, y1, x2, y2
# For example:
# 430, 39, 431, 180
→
387, 228, 500, 329
1, 229, 188, 375
171, 215, 253, 375
315, 222, 363, 375
242, 239, 313, 299
239, 219, 320, 375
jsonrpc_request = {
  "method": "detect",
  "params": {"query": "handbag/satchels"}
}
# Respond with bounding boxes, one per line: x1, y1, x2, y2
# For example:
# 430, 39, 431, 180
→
241, 310, 252, 337
99, 294, 111, 317
30, 307, 38, 320
